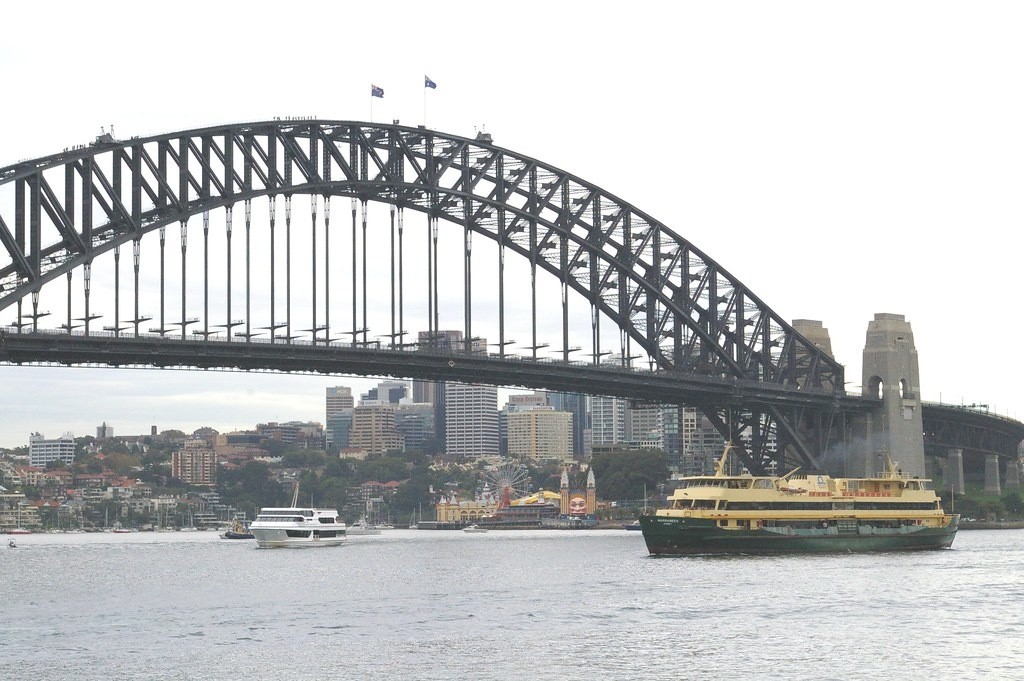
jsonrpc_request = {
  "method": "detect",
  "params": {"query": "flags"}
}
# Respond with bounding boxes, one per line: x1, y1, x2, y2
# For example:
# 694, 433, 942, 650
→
371, 86, 384, 98
424, 76, 436, 89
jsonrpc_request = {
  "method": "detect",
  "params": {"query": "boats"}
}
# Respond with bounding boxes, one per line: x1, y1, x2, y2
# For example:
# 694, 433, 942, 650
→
113, 529, 130, 533
637, 437, 961, 559
225, 520, 255, 539
248, 506, 352, 549
8, 528, 31, 534
